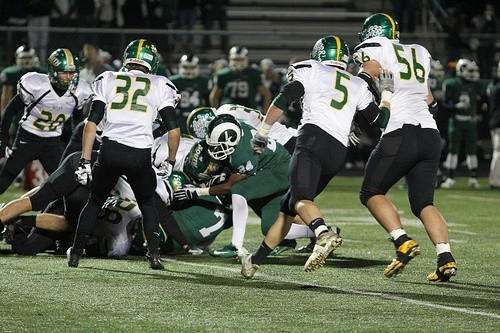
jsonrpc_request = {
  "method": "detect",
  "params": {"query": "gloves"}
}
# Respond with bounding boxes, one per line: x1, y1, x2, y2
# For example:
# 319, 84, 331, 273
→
157, 157, 176, 179
74, 158, 92, 185
250, 130, 268, 153
173, 184, 198, 201
375, 68, 394, 93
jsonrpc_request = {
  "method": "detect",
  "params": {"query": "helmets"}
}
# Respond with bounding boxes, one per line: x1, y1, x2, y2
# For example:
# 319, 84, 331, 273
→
186, 106, 217, 139
124, 38, 159, 74
188, 141, 222, 178
169, 171, 190, 190
358, 13, 399, 41
48, 48, 80, 93
16, 45, 36, 69
311, 35, 349, 70
432, 58, 479, 81
205, 114, 242, 160
179, 46, 273, 79
126, 214, 167, 252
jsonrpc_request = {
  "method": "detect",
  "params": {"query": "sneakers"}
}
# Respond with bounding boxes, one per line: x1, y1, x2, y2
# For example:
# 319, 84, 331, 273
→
427, 256, 457, 281
384, 239, 420, 277
304, 228, 343, 272
0, 203, 296, 279
299, 226, 341, 253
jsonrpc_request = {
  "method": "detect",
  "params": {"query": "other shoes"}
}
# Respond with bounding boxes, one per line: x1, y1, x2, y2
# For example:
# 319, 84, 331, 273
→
469, 178, 478, 188
440, 179, 455, 189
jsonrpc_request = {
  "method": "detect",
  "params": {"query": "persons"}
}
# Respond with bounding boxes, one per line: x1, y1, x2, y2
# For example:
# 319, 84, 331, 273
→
352, 12, 459, 283
0, 45, 500, 255
236, 35, 394, 280
65, 39, 181, 270
